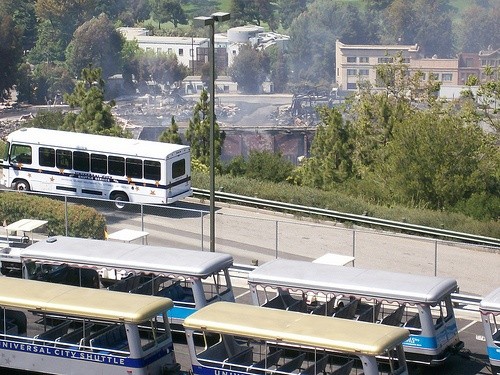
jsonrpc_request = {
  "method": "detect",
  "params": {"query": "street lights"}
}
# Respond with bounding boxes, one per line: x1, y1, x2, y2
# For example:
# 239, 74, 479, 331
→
193, 12, 232, 252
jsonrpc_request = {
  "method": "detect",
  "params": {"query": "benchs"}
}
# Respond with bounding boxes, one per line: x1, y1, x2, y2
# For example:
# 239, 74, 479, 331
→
31, 323, 134, 357
260, 293, 422, 332
102, 271, 194, 304
218, 345, 357, 375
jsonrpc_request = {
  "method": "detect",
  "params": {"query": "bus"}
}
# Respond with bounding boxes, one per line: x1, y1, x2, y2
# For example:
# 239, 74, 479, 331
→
0, 275, 181, 375
0, 234, 43, 278
182, 300, 411, 375
247, 258, 466, 364
1, 127, 193, 211
479, 288, 500, 374
20, 235, 236, 337
